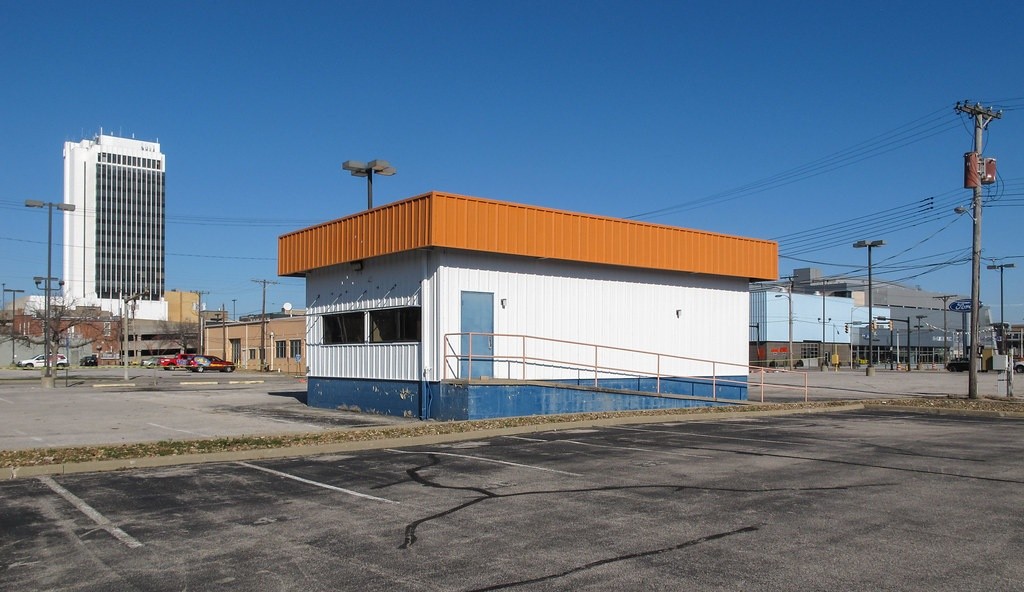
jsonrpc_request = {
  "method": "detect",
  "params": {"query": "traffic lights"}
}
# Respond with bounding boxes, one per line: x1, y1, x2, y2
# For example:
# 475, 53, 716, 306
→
888, 321, 893, 331
845, 324, 848, 334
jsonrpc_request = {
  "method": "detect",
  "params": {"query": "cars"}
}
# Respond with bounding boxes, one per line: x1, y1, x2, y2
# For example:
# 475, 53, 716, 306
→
141, 357, 168, 369
1014, 361, 1024, 373
80, 355, 98, 367
946, 359, 970, 372
186, 355, 235, 373
17, 351, 70, 369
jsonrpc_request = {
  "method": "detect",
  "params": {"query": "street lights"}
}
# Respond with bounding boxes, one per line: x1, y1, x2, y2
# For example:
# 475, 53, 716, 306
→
2, 283, 6, 325
914, 326, 924, 364
818, 318, 831, 365
24, 200, 75, 376
775, 294, 793, 371
342, 159, 398, 210
954, 205, 981, 399
4, 289, 24, 363
853, 239, 888, 365
35, 279, 65, 366
987, 262, 1015, 354
232, 299, 238, 321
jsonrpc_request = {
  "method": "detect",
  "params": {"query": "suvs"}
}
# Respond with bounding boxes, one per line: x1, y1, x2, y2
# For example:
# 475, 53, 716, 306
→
158, 354, 196, 372
